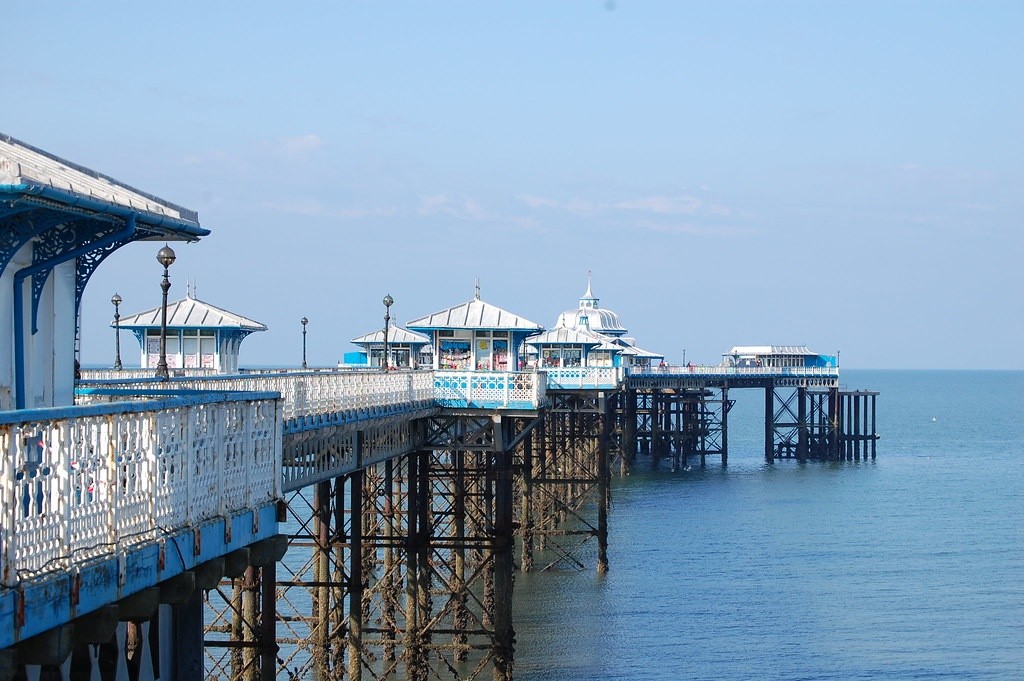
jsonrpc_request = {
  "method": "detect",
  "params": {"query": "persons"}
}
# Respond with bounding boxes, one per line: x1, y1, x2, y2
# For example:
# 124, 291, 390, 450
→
659, 360, 670, 367
686, 361, 691, 367
757, 359, 761, 366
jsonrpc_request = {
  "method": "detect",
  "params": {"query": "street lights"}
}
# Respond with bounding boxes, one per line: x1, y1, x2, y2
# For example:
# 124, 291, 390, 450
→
111, 295, 123, 370
382, 295, 394, 369
156, 246, 177, 376
301, 317, 309, 368
